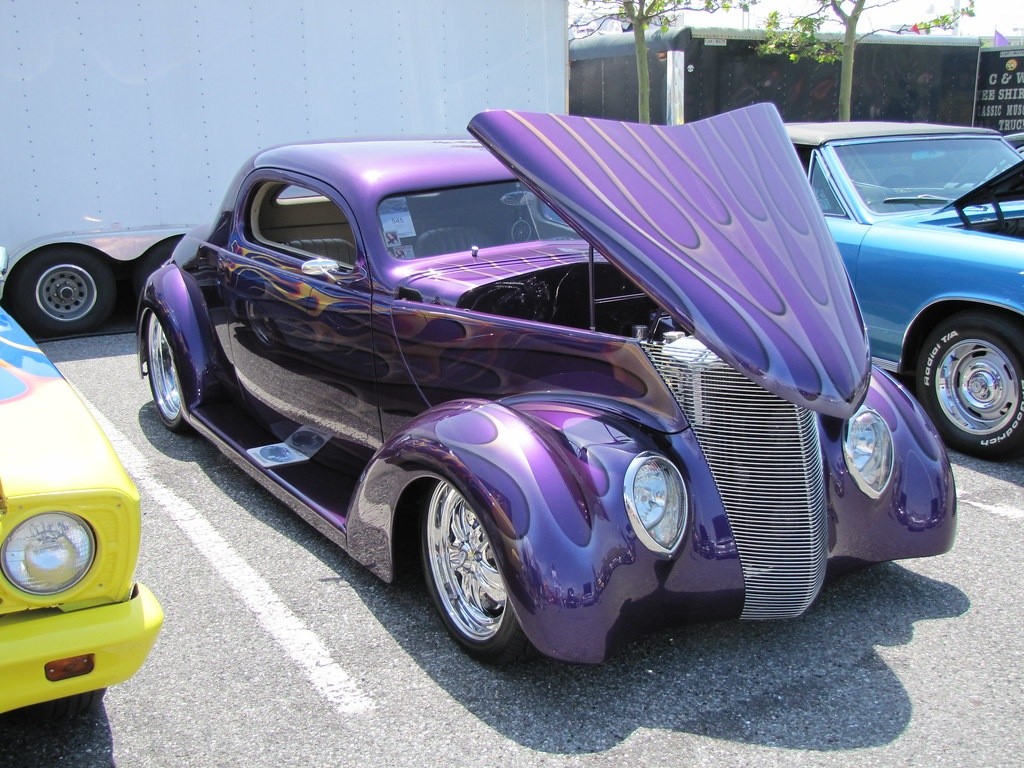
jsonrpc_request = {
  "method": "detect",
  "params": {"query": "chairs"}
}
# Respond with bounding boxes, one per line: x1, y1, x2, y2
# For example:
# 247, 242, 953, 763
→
285, 240, 356, 267
415, 225, 494, 259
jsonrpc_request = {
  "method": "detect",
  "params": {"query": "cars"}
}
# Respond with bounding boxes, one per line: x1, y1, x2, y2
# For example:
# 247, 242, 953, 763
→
0, 302, 165, 735
136, 100, 962, 680
777, 117, 1024, 465
940, 129, 1023, 204
